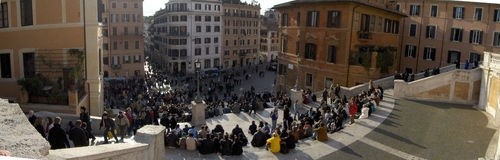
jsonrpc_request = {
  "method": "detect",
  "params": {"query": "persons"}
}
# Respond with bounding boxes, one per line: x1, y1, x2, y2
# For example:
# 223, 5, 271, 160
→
25, 53, 478, 155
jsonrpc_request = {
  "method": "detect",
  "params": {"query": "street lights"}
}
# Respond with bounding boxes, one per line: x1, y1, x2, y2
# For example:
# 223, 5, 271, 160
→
194, 58, 202, 103
292, 53, 302, 91
